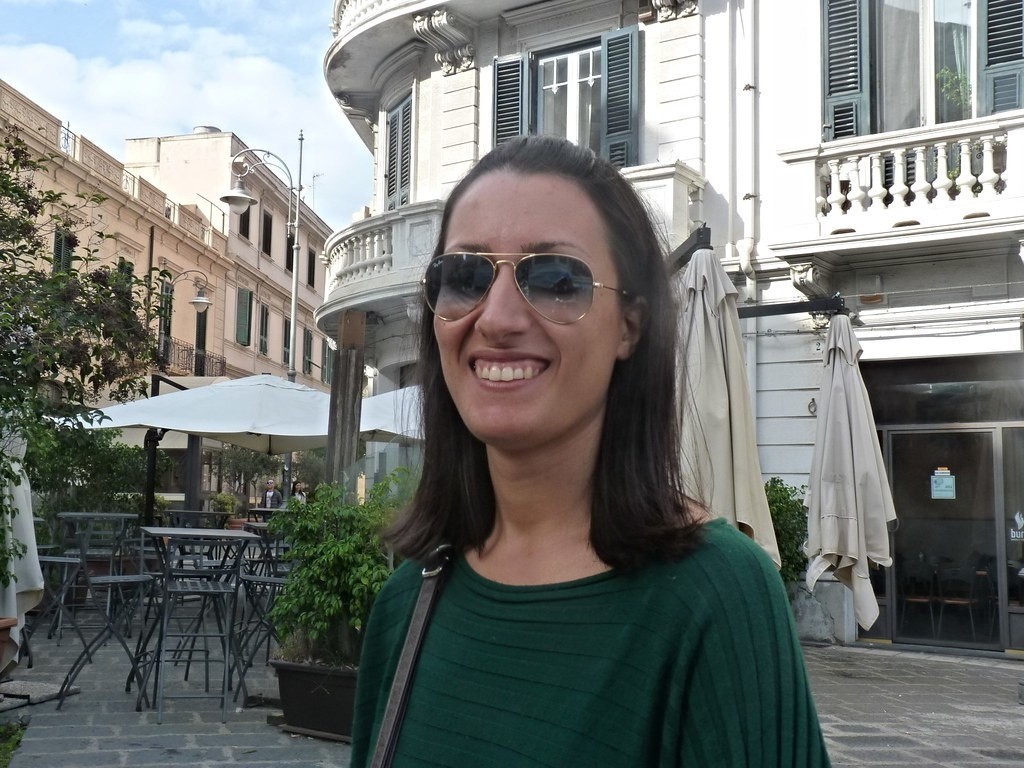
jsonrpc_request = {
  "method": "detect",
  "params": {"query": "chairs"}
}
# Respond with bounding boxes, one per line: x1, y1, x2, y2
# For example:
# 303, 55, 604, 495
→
899, 550, 1019, 643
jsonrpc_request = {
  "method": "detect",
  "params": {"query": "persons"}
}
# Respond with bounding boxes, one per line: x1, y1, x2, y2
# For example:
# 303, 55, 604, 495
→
348, 134, 832, 767
259, 480, 283, 519
290, 480, 307, 508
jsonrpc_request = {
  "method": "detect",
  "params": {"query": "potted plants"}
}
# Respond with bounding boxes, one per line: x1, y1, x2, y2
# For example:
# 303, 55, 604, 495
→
0, 460, 29, 666
263, 464, 418, 747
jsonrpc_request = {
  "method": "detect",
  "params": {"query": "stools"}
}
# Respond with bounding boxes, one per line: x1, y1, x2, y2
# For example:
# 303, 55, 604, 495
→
16, 526, 296, 726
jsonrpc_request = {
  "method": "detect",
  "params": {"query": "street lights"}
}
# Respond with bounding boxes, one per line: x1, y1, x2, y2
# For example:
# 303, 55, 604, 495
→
157, 256, 213, 377
220, 127, 306, 385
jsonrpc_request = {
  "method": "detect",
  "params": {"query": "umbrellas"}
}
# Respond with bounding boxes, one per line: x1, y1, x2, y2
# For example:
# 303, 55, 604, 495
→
969, 376, 1024, 559
669, 249, 780, 569
354, 378, 428, 443
41, 373, 330, 457
1, 404, 44, 680
803, 314, 894, 635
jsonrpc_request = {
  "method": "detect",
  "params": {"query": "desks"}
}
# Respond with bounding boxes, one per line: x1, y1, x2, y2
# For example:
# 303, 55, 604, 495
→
165, 510, 236, 528
57, 513, 138, 640
251, 509, 301, 520
244, 522, 271, 529
140, 530, 261, 723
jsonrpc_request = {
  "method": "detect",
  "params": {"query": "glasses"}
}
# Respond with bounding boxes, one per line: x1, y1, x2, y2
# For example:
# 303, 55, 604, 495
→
420, 251, 635, 325
266, 481, 274, 484
